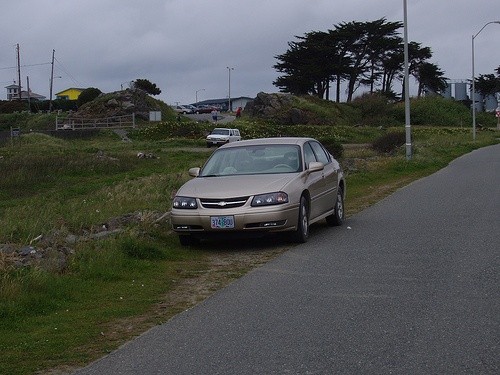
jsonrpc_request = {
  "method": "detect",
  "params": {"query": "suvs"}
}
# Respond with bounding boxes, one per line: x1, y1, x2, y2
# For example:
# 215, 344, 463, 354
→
205, 128, 242, 148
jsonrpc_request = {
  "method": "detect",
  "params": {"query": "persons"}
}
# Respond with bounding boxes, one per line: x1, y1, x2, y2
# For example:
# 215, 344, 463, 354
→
212, 109, 217, 121
177, 114, 180, 121
236, 107, 242, 118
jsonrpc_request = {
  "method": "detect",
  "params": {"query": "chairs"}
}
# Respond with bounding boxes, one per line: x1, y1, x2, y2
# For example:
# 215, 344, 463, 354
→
231, 151, 298, 172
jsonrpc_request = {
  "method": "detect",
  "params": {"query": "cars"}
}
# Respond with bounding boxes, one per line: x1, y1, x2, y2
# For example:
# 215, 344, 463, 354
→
173, 103, 221, 115
170, 137, 347, 247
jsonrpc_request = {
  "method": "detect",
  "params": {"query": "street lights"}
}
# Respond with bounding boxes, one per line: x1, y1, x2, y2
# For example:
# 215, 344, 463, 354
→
195, 88, 205, 106
49, 76, 62, 90
226, 66, 235, 112
472, 21, 500, 142
